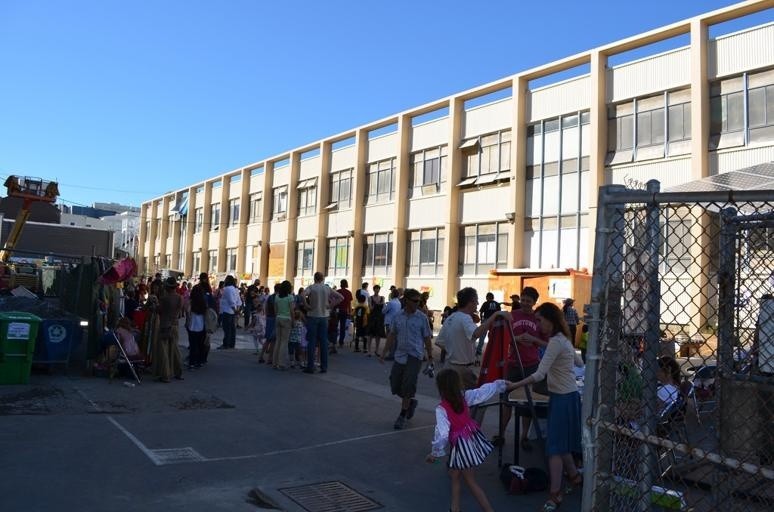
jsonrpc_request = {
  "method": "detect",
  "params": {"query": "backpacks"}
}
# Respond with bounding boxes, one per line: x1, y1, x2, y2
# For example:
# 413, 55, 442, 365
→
204, 308, 219, 339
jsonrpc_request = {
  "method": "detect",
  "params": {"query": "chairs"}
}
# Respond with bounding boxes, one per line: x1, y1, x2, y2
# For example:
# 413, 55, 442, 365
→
685, 364, 721, 439
619, 378, 696, 478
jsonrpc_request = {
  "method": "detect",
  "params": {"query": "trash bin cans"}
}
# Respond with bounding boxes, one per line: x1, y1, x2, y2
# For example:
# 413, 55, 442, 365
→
0, 310, 43, 386
31, 312, 82, 375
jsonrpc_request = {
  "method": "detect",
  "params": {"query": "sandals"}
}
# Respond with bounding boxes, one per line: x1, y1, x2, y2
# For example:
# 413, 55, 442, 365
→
535, 490, 563, 512
563, 471, 582, 496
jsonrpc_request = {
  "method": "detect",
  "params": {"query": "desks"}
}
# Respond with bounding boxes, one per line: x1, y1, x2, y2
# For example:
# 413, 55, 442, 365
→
508, 383, 584, 476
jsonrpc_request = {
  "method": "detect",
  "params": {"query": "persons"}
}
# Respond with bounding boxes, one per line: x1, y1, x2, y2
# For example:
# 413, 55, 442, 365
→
214, 275, 269, 350
425, 367, 514, 511
613, 355, 684, 478
378, 286, 436, 432
490, 285, 549, 453
420, 288, 591, 391
250, 272, 404, 375
502, 300, 583, 511
97, 268, 213, 381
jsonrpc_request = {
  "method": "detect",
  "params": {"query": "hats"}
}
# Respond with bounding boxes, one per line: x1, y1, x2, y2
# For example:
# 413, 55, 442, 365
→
166, 277, 179, 288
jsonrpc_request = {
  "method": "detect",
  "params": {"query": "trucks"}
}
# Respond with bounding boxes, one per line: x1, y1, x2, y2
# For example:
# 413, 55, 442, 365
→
484, 266, 643, 374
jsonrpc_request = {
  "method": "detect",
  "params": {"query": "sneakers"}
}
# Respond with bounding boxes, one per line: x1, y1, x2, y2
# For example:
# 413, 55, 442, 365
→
393, 398, 417, 431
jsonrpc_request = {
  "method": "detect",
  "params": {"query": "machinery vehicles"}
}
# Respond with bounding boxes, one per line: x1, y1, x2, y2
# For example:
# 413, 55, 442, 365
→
0, 174, 60, 298
